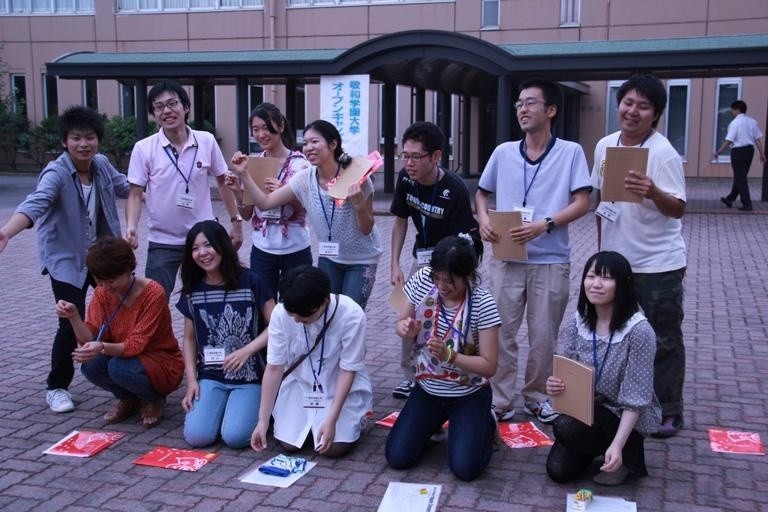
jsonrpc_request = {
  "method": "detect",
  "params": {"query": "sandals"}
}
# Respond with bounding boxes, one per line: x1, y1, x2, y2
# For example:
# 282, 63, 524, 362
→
102, 399, 134, 424
139, 402, 166, 429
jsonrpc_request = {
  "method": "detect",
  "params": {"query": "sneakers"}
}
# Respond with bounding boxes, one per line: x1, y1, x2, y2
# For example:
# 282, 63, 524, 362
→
594, 465, 629, 486
491, 405, 517, 423
391, 378, 414, 400
651, 412, 684, 438
522, 396, 561, 423
45, 389, 75, 413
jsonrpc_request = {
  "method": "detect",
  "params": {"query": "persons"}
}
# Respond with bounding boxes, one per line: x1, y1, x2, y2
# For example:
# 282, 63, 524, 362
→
718, 100, 766, 211
590, 75, 687, 438
224, 102, 311, 304
0, 105, 146, 413
250, 264, 374, 459
231, 120, 383, 310
546, 251, 663, 486
474, 76, 593, 424
125, 79, 243, 305
54, 236, 185, 429
175, 220, 276, 450
384, 232, 497, 482
390, 122, 483, 399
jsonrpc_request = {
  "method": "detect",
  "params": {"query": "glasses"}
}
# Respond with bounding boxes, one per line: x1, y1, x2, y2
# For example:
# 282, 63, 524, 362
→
513, 98, 545, 110
151, 97, 179, 111
399, 149, 429, 162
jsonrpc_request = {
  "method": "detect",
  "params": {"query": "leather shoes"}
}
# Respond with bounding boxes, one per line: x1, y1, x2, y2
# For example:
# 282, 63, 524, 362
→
721, 197, 732, 208
738, 205, 752, 211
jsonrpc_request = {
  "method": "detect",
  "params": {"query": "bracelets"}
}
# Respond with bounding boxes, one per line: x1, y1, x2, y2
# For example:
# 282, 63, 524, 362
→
233, 199, 246, 208
445, 344, 457, 365
545, 217, 555, 234
716, 150, 720, 155
101, 342, 105, 355
230, 213, 242, 223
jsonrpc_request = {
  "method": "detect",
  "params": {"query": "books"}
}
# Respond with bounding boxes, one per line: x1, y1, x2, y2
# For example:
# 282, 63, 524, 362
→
601, 147, 649, 204
242, 157, 280, 205
551, 354, 595, 427
487, 209, 528, 261
326, 150, 385, 207
